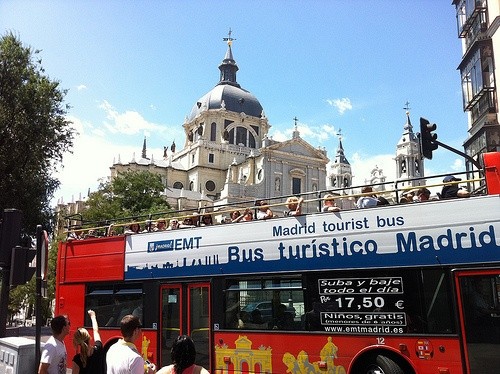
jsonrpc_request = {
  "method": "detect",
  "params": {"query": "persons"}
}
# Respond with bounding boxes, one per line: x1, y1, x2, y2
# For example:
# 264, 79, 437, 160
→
67, 209, 253, 242
105, 314, 157, 374
155, 335, 211, 374
441, 176, 472, 198
72, 310, 106, 374
38, 315, 71, 374
356, 185, 431, 209
472, 286, 494, 318
283, 196, 303, 217
321, 194, 341, 211
234, 311, 272, 328
255, 200, 278, 220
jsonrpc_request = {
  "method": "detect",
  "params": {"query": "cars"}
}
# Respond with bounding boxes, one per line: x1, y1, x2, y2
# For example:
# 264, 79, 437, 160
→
243, 302, 296, 323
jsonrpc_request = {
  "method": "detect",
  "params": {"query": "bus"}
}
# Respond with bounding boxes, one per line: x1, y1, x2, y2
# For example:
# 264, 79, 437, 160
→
53, 151, 500, 374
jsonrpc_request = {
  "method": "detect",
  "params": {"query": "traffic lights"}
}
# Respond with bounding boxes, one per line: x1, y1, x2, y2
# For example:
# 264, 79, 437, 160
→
419, 117, 438, 160
9, 245, 36, 290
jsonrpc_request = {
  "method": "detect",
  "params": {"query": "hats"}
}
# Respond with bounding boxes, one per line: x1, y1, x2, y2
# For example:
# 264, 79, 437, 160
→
442, 176, 461, 182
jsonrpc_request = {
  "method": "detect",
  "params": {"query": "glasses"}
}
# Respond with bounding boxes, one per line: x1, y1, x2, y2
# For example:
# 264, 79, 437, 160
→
159, 222, 166, 224
65, 321, 70, 326
137, 323, 143, 329
203, 216, 211, 220
184, 219, 192, 222
326, 199, 334, 202
